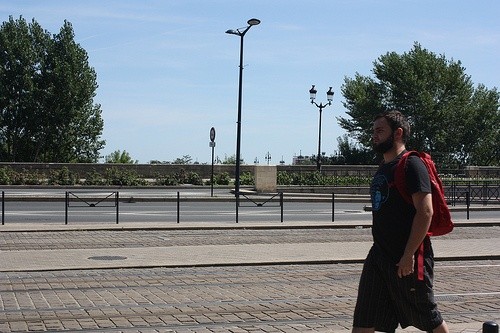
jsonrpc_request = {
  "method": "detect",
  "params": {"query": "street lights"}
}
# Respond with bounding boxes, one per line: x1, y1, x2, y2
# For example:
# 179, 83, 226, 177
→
308, 85, 334, 173
226, 19, 260, 199
264, 152, 271, 166
280, 159, 285, 165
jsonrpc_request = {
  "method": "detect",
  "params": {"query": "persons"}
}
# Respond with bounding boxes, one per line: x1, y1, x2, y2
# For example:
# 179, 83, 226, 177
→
351, 110, 451, 333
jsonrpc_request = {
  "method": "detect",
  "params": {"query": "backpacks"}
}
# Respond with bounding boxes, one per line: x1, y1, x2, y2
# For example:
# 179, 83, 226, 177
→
395, 150, 455, 237
